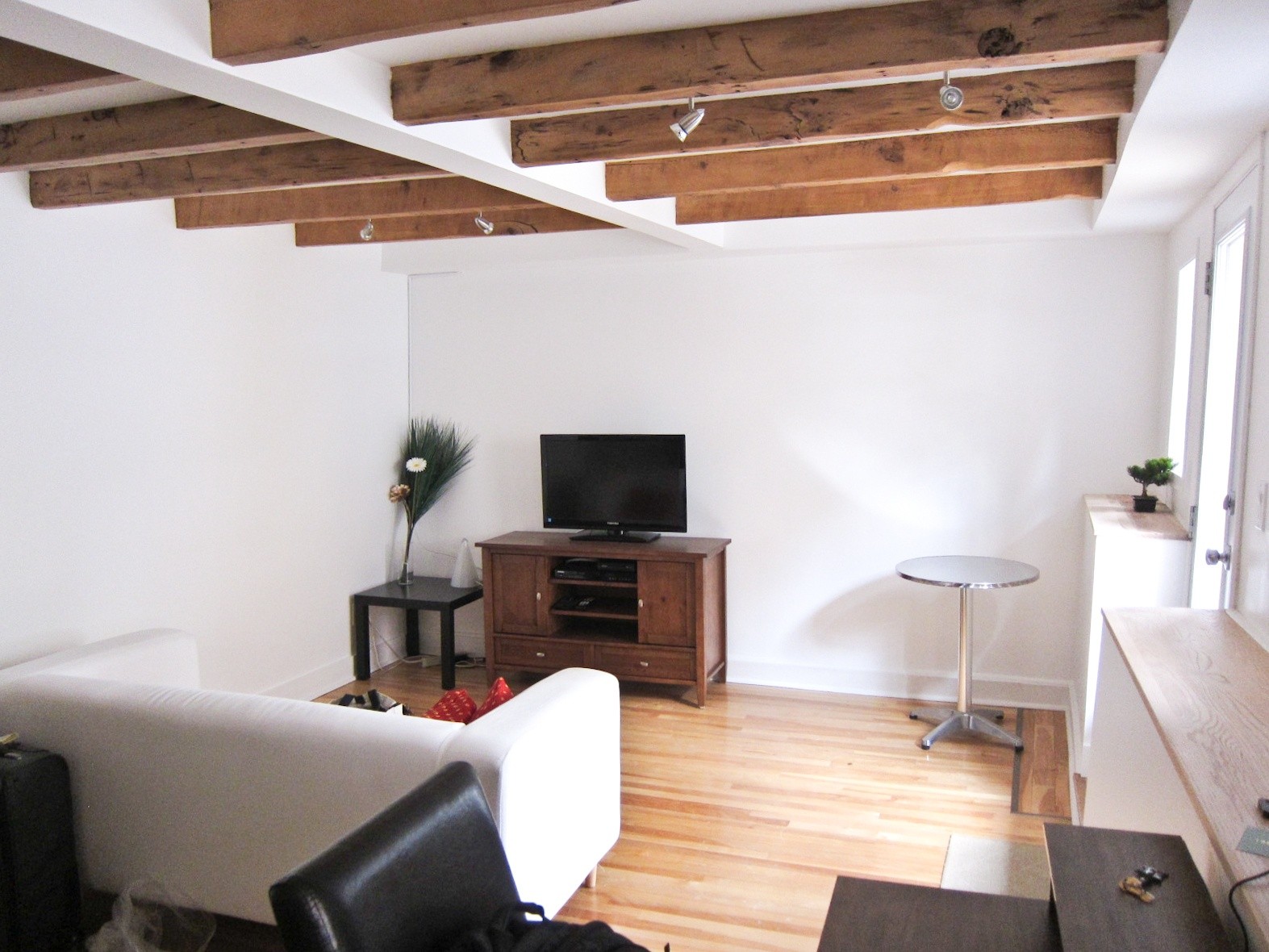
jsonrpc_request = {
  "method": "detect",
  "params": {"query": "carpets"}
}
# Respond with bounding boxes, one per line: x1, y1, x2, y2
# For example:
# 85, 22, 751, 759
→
940, 834, 1051, 902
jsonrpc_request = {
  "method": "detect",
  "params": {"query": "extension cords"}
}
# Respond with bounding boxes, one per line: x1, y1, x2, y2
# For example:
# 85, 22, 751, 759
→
422, 651, 468, 667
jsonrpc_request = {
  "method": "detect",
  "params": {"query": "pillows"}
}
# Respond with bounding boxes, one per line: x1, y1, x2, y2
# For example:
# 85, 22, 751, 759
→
426, 688, 477, 725
468, 676, 515, 722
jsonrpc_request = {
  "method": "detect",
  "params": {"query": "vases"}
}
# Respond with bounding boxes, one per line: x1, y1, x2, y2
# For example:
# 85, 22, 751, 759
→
396, 562, 414, 586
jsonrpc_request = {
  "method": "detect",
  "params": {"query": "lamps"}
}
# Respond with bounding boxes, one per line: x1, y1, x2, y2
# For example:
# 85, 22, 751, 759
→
474, 212, 494, 235
939, 71, 964, 111
359, 218, 374, 240
669, 98, 705, 142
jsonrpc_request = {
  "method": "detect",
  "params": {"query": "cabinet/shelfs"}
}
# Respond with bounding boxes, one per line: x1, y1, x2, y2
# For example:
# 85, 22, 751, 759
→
817, 821, 1233, 952
475, 531, 733, 709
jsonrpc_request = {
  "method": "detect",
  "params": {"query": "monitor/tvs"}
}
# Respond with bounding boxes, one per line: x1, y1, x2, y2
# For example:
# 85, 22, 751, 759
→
540, 435, 688, 542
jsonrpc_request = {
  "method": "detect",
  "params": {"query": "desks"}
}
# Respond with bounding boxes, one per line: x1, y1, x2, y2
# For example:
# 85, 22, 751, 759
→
353, 576, 483, 691
1080, 608, 1269, 952
895, 556, 1040, 755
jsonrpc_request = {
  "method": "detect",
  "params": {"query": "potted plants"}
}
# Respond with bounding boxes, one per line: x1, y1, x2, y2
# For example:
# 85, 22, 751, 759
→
1127, 457, 1175, 513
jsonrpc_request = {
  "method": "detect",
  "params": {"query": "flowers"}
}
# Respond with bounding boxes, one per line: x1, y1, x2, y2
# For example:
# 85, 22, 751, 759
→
388, 413, 475, 584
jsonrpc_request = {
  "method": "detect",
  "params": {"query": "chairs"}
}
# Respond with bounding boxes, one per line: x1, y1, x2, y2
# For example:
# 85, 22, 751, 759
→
268, 762, 653, 952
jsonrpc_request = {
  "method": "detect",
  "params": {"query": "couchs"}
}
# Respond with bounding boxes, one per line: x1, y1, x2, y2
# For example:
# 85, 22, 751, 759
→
0, 626, 621, 929
